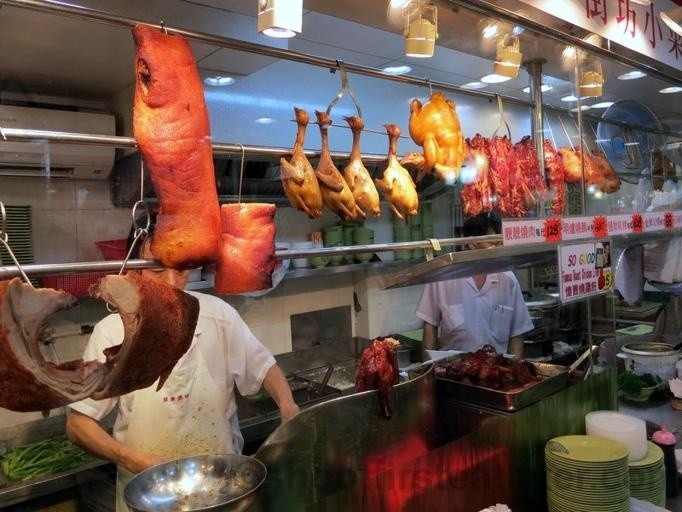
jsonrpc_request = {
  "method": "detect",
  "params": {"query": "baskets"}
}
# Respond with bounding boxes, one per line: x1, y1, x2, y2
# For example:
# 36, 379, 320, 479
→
95, 238, 131, 259
39, 271, 128, 296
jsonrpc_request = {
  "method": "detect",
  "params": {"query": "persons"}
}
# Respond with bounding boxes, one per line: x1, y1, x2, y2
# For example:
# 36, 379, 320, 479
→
414, 214, 536, 365
65, 208, 301, 511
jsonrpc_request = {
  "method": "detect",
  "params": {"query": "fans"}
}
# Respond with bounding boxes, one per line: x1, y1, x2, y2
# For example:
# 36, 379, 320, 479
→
596, 100, 665, 186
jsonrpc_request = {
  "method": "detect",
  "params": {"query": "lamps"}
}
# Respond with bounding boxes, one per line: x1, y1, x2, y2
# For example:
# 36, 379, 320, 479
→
402, 1, 438, 58
494, 32, 523, 79
659, 6, 682, 36
257, 1, 304, 39
575, 58, 604, 97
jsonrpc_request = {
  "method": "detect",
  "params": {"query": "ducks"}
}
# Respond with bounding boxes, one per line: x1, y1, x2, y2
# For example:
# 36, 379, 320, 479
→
313, 109, 368, 222
280, 105, 326, 219
341, 115, 383, 218
373, 123, 419, 221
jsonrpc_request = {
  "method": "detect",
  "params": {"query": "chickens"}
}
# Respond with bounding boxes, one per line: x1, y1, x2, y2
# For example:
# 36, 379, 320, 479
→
399, 91, 465, 184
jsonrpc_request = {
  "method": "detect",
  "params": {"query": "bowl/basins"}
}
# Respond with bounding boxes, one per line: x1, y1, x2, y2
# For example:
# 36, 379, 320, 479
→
273, 242, 315, 269
397, 345, 415, 368
624, 383, 661, 402
309, 223, 374, 269
393, 201, 446, 261
123, 454, 268, 512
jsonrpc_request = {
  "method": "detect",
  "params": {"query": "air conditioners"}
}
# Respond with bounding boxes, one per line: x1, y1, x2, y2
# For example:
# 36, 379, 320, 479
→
0, 102, 116, 180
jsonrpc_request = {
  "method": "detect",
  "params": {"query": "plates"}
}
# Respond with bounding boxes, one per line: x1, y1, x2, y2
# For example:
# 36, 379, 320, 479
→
0, 204, 39, 288
545, 411, 667, 512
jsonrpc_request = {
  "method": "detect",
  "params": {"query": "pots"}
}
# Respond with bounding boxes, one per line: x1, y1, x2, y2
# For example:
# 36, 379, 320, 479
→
616, 341, 680, 384
522, 290, 560, 358
